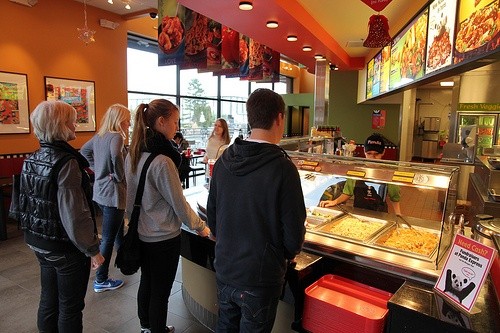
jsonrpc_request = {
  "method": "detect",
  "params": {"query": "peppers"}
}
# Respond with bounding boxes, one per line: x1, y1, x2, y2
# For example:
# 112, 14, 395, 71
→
222, 27, 240, 63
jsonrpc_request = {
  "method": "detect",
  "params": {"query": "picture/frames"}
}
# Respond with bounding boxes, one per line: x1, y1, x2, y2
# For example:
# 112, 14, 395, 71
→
44, 76, 97, 132
0, 71, 31, 134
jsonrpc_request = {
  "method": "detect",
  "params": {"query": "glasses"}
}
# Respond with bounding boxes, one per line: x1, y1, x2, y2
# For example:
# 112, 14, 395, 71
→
214, 124, 222, 127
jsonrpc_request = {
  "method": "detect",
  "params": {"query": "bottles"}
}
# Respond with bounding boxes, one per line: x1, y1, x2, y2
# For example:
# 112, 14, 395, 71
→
317, 125, 341, 138
335, 147, 340, 155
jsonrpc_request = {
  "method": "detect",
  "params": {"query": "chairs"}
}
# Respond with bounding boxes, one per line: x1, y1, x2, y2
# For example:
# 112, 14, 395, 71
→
189, 149, 207, 178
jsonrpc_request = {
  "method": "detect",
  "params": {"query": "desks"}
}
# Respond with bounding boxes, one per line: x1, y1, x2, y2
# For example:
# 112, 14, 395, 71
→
0, 178, 15, 241
184, 153, 204, 186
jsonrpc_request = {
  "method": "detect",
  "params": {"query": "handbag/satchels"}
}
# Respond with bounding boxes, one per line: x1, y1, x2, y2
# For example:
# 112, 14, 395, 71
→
9, 173, 22, 221
114, 229, 144, 275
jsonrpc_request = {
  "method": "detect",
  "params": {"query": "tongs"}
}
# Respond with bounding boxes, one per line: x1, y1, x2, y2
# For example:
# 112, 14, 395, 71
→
396, 214, 412, 234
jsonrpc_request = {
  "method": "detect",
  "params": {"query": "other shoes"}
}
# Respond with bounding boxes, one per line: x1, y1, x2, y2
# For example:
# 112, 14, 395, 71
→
140, 324, 175, 333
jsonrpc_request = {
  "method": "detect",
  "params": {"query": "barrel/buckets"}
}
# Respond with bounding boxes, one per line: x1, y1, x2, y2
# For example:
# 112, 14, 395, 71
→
474, 218, 500, 252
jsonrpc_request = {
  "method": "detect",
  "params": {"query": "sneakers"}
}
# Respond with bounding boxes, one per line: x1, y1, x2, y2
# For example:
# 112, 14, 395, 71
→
94, 278, 125, 293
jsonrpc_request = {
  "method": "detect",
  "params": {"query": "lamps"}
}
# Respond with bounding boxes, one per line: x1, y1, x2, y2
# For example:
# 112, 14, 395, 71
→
76, 0, 95, 45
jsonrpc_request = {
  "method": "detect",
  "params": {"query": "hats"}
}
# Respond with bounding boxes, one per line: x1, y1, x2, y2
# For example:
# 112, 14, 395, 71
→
365, 135, 385, 154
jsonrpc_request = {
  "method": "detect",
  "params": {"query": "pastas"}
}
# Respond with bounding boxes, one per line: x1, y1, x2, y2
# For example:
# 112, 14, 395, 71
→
250, 39, 268, 63
184, 14, 213, 54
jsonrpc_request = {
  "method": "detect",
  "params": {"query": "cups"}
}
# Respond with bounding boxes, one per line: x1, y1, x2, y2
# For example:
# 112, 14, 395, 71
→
187, 148, 191, 156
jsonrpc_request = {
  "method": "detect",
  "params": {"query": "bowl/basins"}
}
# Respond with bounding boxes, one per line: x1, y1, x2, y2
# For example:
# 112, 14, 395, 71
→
185, 48, 207, 61
240, 59, 248, 71
158, 20, 186, 55
250, 63, 263, 75
455, 27, 500, 57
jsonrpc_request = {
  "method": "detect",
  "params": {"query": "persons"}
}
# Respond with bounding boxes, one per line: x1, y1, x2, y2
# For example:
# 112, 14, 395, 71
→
78, 103, 131, 292
320, 134, 401, 217
170, 132, 190, 183
18, 101, 105, 333
202, 118, 230, 182
206, 88, 307, 333
124, 99, 210, 333
